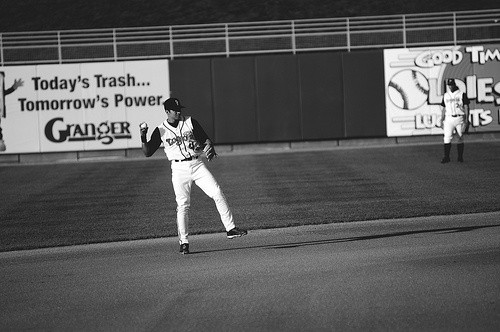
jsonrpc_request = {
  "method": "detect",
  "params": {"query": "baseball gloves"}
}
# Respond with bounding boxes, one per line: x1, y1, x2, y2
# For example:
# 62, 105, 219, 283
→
203, 138, 215, 162
461, 120, 471, 134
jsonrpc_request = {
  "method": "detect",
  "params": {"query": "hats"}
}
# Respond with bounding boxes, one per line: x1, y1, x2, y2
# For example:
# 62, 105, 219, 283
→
448, 79, 456, 86
163, 98, 182, 110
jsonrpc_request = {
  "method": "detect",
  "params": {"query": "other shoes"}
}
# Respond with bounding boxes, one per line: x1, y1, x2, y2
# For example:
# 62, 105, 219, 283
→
441, 158, 451, 163
458, 159, 464, 163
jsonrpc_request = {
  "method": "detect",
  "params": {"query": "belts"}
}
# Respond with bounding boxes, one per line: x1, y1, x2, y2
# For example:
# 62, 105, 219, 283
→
175, 156, 198, 162
452, 115, 461, 117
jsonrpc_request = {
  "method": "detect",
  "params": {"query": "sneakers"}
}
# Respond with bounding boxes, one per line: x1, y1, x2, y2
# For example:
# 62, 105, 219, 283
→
180, 243, 189, 254
227, 227, 247, 239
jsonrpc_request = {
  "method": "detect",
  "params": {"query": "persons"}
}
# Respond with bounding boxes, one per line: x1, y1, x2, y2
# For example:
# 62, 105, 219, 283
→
139, 98, 247, 254
440, 78, 470, 164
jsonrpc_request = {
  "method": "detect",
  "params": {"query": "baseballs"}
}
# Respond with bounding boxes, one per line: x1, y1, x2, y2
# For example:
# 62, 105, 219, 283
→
141, 123, 147, 128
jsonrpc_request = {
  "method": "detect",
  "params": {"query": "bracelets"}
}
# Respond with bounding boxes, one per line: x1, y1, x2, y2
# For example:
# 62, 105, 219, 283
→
141, 136, 146, 144
